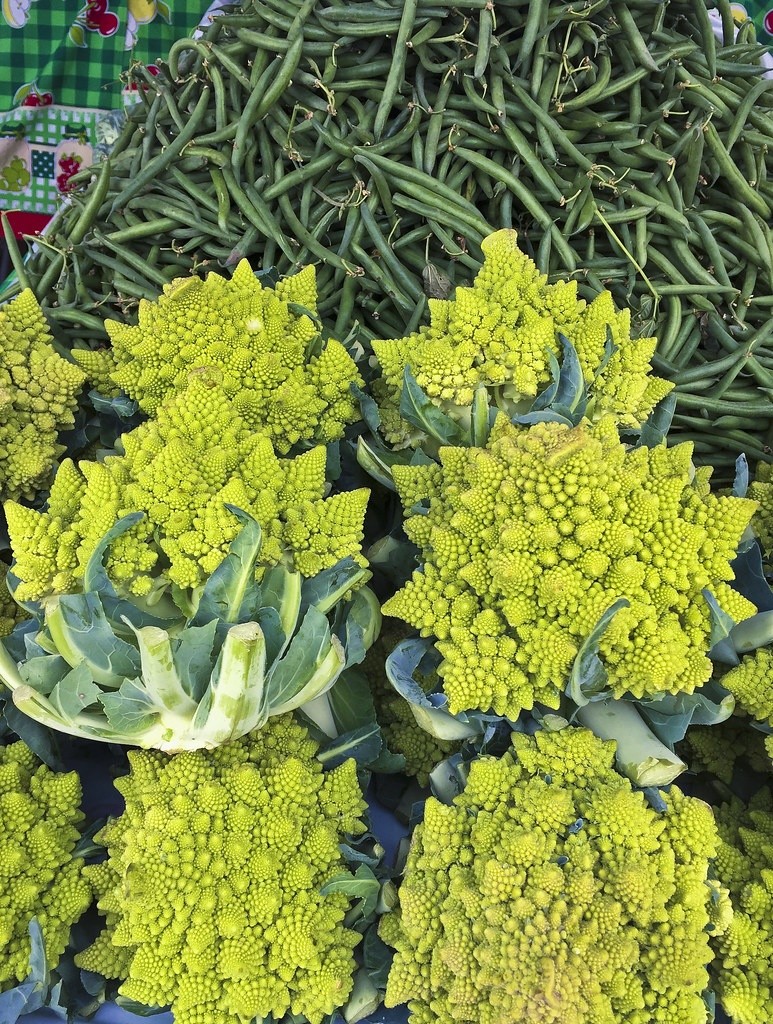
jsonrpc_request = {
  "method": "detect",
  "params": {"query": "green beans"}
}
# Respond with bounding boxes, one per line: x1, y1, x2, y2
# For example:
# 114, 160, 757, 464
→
0, 0, 773, 487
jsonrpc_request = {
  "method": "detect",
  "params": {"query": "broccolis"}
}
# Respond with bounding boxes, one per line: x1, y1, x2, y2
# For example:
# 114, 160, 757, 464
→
0, 233, 773, 1024
